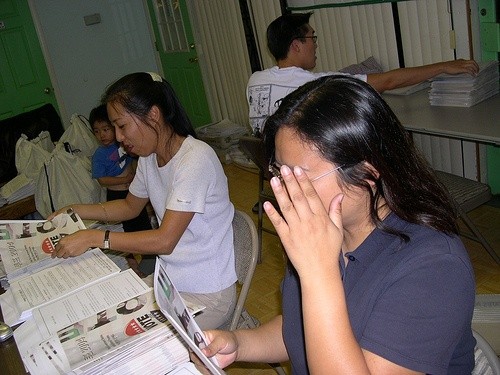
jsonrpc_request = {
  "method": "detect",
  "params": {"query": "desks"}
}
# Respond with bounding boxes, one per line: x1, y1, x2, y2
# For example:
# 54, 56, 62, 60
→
383, 62, 500, 151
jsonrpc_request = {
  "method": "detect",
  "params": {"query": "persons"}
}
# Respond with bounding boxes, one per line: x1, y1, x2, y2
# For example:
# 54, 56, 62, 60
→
46, 71, 239, 331
189, 74, 477, 375
88, 105, 155, 279
246, 10, 478, 137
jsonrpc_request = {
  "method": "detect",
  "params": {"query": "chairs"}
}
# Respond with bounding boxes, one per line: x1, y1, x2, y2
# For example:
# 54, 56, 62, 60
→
422, 155, 500, 269
238, 132, 280, 267
219, 209, 286, 375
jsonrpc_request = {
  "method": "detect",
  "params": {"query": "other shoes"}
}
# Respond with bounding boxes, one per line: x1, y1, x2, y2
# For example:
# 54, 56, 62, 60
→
135, 255, 154, 278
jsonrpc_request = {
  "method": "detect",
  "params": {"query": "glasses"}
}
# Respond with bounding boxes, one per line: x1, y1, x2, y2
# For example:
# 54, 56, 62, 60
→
269, 148, 346, 189
288, 36, 317, 47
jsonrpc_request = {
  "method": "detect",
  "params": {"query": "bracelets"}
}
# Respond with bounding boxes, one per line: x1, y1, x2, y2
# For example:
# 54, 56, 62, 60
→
103, 230, 110, 250
96, 201, 108, 223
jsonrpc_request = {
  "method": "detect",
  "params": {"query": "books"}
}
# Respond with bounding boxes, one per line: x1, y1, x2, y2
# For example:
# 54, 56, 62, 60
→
338, 56, 429, 96
426, 61, 499, 108
0, 208, 226, 375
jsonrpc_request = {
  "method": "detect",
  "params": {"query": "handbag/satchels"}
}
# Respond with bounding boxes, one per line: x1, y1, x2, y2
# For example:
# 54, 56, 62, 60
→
15, 113, 101, 219
